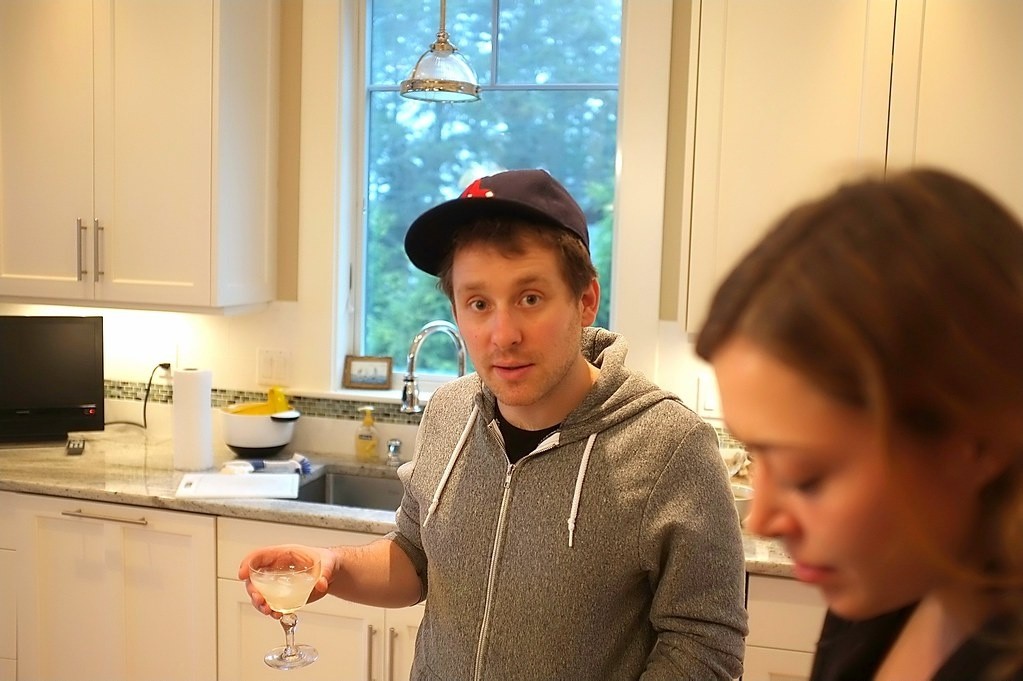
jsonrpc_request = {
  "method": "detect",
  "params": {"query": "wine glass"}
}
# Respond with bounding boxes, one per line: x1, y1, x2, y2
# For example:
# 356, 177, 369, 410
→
250, 545, 323, 671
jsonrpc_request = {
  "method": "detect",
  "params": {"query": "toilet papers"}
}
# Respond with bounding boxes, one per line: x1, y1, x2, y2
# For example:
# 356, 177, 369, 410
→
172, 368, 211, 471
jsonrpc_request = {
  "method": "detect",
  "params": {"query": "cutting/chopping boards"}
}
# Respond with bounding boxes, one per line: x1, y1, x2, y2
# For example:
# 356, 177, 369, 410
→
174, 472, 300, 499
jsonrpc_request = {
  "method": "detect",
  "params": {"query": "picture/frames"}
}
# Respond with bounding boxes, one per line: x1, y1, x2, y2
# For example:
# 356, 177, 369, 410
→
343, 355, 393, 390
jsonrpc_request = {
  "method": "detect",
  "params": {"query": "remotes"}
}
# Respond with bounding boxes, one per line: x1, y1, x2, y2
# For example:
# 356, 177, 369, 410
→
66, 439, 86, 456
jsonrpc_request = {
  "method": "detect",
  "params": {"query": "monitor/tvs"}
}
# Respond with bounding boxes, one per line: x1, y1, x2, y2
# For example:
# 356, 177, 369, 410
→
0, 316, 105, 445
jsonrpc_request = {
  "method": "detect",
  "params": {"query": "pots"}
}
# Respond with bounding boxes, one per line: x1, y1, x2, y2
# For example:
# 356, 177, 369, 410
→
221, 400, 300, 459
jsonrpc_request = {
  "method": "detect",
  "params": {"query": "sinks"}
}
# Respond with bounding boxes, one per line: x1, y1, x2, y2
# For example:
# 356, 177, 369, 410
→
267, 464, 397, 512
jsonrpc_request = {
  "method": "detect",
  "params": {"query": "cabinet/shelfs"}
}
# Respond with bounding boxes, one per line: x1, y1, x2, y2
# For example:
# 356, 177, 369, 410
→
216, 516, 426, 681
659, 0, 1023, 344
0, 0, 304, 314
0, 489, 217, 681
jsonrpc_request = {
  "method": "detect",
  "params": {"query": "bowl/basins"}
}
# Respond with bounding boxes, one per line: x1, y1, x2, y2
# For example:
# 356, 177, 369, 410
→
730, 485, 755, 525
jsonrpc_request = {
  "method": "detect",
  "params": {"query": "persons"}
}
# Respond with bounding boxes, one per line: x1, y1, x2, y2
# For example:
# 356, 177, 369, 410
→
239, 169, 750, 681
694, 164, 1023, 681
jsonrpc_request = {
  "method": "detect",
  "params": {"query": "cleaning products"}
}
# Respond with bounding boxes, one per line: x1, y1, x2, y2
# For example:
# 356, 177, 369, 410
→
354, 405, 379, 464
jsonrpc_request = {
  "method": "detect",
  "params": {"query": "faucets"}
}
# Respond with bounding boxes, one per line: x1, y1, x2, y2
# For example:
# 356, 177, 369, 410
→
386, 439, 403, 468
400, 320, 467, 414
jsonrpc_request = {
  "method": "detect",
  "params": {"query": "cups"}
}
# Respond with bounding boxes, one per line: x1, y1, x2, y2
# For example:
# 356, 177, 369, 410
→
719, 449, 746, 483
718, 431, 748, 449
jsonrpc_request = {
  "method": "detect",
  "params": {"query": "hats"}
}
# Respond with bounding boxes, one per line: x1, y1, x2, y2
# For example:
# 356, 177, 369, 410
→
405, 170, 591, 277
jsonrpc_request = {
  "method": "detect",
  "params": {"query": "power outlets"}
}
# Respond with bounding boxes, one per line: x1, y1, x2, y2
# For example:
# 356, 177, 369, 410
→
255, 349, 295, 387
157, 345, 177, 378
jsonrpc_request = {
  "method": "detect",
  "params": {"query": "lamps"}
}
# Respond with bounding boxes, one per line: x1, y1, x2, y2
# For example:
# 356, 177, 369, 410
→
400, 0, 482, 103
